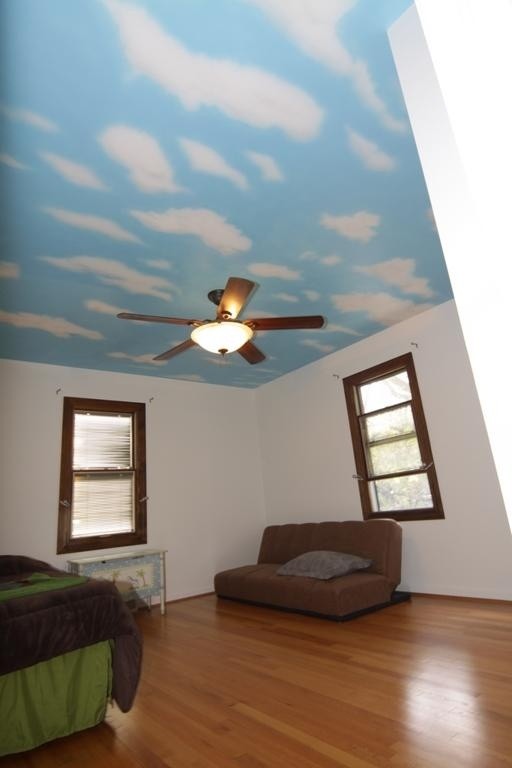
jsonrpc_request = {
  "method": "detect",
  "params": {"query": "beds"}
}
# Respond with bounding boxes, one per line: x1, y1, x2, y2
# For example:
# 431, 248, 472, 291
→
1, 551, 141, 758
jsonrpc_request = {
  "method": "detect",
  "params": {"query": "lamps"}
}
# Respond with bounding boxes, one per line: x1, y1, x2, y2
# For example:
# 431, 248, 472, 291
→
189, 318, 252, 358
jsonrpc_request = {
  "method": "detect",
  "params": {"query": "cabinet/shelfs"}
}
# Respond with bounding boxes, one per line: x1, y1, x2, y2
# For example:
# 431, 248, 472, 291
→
67, 549, 168, 618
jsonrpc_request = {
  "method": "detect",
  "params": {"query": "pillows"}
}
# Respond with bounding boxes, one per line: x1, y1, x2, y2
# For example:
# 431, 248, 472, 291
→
274, 547, 373, 582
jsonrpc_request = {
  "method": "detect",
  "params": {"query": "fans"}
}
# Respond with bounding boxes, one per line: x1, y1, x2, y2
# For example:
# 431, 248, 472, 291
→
116, 272, 324, 366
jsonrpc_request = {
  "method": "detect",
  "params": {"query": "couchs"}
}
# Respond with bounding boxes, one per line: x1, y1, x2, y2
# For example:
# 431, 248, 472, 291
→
214, 518, 401, 616
